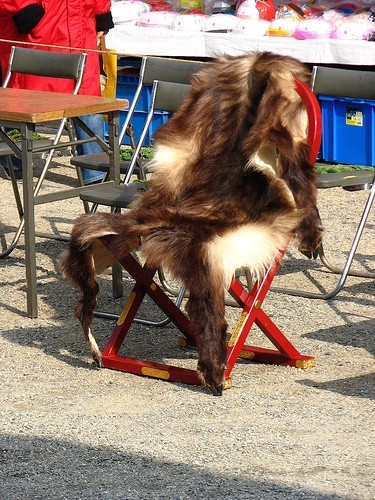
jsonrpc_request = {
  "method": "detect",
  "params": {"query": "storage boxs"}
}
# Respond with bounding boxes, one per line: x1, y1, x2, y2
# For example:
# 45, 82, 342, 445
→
319, 94, 375, 166
103, 74, 169, 148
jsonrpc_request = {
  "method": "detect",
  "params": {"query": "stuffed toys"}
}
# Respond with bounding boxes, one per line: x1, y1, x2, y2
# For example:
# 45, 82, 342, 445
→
110, 0, 375, 41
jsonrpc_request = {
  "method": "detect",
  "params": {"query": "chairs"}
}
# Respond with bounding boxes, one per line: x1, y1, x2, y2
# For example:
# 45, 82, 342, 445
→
0, 45, 375, 395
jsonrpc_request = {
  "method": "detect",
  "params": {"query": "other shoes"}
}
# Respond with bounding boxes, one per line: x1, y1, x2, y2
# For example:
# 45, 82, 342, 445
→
3, 163, 22, 180
82, 178, 125, 187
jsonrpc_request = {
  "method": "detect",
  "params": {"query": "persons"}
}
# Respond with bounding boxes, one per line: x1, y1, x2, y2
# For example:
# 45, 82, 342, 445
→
0, 0, 115, 186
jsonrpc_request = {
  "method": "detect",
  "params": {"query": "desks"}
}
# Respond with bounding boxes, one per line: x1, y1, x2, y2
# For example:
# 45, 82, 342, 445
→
0, 87, 128, 318
